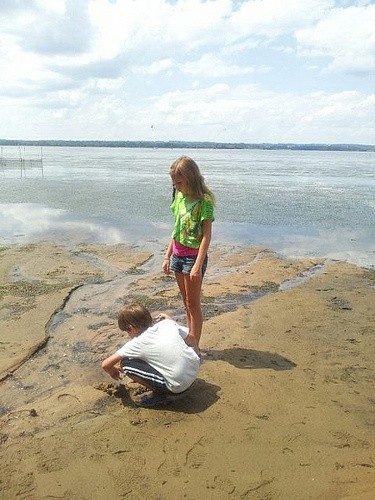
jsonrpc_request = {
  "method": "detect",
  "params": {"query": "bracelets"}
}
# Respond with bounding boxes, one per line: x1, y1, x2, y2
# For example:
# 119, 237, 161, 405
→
164, 257, 169, 260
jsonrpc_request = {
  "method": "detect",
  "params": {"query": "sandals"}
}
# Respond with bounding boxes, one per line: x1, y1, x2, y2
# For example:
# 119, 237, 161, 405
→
134, 390, 180, 406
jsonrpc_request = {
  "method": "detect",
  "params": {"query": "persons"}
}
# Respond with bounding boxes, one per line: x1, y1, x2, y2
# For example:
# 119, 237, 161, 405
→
162, 156, 215, 357
101, 303, 201, 406
